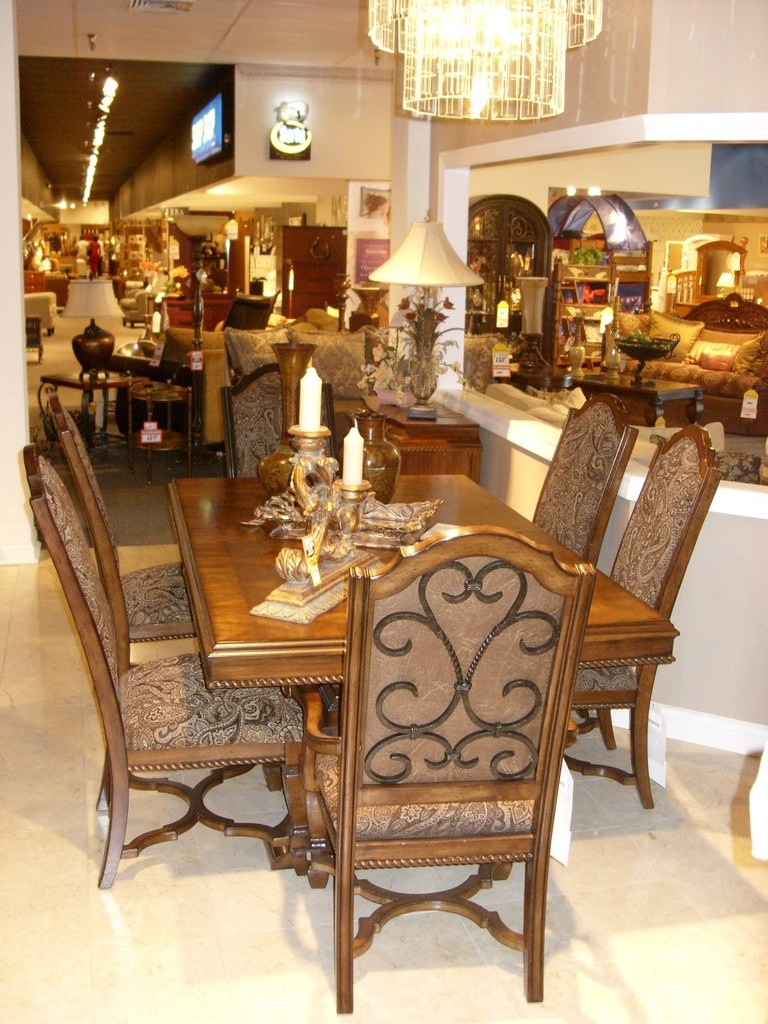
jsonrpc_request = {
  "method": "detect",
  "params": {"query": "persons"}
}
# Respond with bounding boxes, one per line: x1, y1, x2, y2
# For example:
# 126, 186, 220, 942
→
87, 237, 101, 281
70, 233, 82, 257
25, 244, 61, 272
104, 236, 121, 276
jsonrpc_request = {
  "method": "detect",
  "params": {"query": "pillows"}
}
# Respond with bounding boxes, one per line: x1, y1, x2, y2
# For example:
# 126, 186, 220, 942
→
223, 326, 290, 381
286, 328, 369, 398
733, 329, 767, 375
647, 310, 706, 362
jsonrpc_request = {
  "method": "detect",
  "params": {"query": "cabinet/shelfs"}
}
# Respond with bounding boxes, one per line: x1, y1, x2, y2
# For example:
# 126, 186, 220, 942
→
24, 269, 46, 294
127, 378, 192, 484
358, 395, 481, 486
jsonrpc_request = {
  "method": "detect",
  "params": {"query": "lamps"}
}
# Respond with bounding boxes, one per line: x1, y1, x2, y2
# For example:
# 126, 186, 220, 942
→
174, 215, 237, 353
367, 0, 602, 121
365, 210, 485, 423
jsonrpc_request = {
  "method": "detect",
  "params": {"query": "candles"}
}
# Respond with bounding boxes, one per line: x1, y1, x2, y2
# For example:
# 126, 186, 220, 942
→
298, 365, 322, 432
342, 416, 365, 485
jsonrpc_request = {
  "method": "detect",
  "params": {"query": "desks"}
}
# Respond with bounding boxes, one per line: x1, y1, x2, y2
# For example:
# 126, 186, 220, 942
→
163, 468, 680, 893
560, 373, 707, 429
36, 372, 153, 453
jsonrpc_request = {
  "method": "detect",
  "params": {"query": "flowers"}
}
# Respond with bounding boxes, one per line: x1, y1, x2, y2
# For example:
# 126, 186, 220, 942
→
356, 327, 417, 402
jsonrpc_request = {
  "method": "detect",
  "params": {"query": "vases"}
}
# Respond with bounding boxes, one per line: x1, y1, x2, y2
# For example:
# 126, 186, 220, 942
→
567, 316, 587, 376
375, 389, 415, 407
350, 409, 402, 504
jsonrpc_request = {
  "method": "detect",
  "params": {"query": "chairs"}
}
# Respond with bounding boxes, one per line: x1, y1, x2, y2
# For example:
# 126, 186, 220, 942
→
23, 441, 332, 893
117, 274, 169, 326
42, 384, 196, 678
560, 422, 723, 810
294, 524, 594, 1014
532, 392, 639, 574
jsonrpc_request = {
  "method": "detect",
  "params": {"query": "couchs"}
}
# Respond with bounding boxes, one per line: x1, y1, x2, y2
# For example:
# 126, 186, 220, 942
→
616, 303, 768, 435
160, 306, 390, 456
